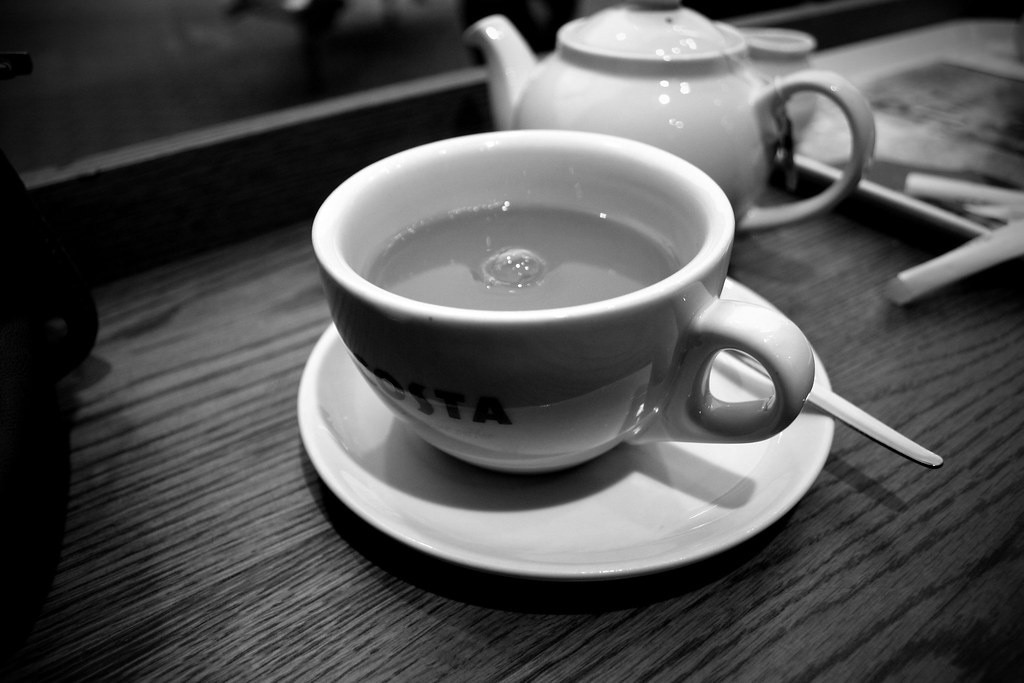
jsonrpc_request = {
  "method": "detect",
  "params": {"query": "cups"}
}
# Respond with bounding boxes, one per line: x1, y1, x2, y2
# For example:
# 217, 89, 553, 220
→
311, 131, 814, 475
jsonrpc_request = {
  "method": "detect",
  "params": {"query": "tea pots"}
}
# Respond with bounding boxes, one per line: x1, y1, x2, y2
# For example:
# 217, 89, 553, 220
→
463, 0, 877, 235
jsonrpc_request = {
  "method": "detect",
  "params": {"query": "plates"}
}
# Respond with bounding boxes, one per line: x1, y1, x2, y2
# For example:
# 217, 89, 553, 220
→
296, 276, 837, 580
